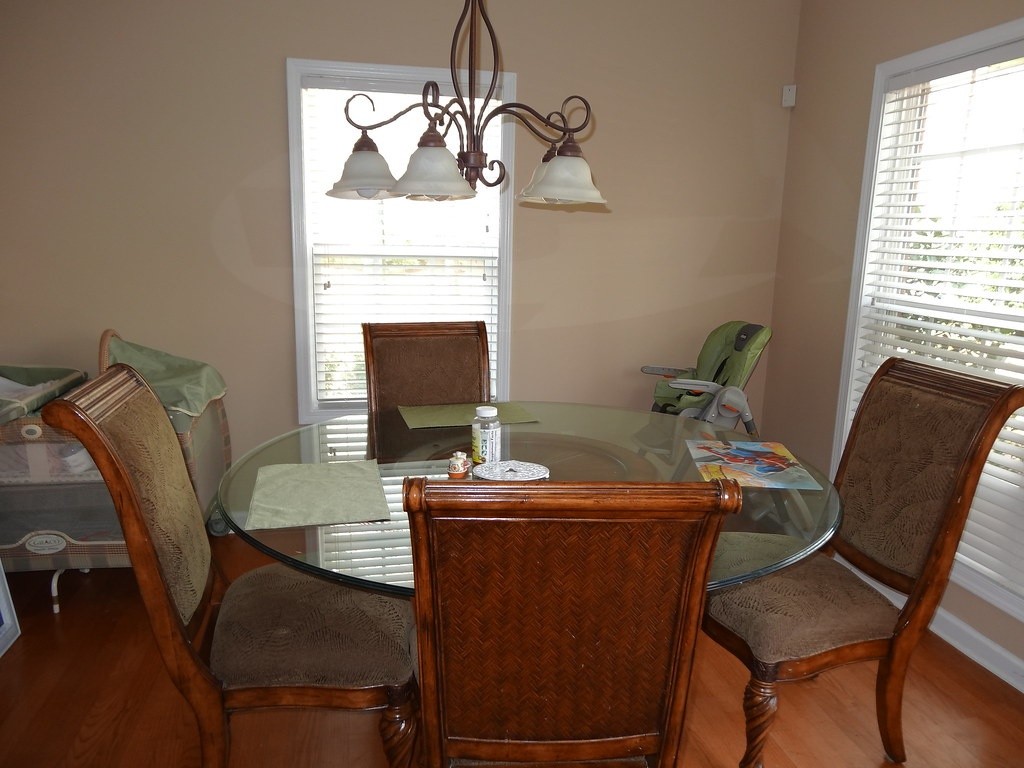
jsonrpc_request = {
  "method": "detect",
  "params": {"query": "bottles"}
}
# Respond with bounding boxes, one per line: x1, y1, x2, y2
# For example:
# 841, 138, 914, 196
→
472, 406, 502, 463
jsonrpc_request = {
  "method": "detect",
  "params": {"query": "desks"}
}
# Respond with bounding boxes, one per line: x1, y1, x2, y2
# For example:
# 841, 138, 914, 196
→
214, 401, 842, 599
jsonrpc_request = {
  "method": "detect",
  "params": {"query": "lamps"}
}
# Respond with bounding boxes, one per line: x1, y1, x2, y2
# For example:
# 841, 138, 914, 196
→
320, 0, 608, 207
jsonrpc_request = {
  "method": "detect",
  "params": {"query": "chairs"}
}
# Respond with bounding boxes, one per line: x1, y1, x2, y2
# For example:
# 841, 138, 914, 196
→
362, 321, 490, 411
41, 362, 423, 767
401, 477, 743, 768
631, 321, 798, 535
700, 357, 1023, 768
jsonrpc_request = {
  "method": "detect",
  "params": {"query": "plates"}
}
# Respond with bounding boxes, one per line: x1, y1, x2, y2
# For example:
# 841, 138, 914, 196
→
472, 460, 550, 481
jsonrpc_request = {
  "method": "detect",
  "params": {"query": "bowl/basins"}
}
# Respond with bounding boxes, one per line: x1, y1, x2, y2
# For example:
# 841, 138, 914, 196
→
446, 462, 471, 479
